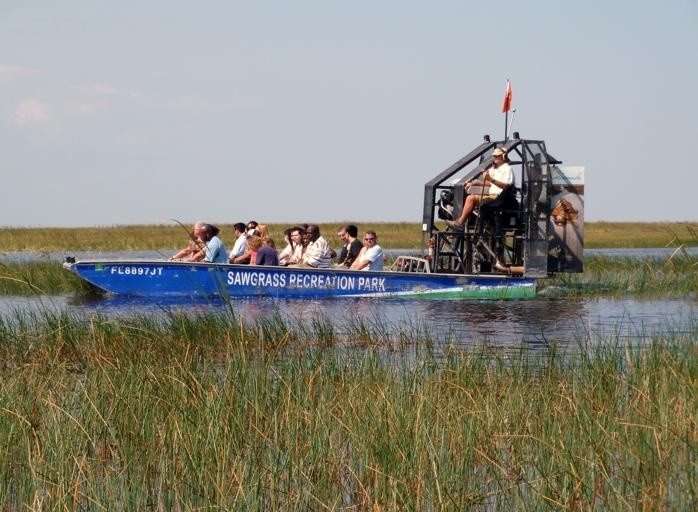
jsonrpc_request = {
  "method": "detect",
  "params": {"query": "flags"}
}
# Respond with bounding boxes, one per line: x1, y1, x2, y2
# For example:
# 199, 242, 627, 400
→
501, 78, 513, 113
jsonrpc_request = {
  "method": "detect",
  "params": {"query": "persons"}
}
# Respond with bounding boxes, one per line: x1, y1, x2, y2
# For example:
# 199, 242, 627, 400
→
442, 146, 515, 233
335, 225, 363, 268
166, 219, 338, 270
333, 225, 351, 266
350, 230, 385, 272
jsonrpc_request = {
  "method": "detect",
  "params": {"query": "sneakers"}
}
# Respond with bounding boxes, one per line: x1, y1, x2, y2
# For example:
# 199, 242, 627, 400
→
445, 219, 465, 232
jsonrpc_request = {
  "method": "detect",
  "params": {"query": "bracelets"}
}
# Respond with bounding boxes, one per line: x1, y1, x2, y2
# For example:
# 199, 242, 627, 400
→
492, 179, 496, 184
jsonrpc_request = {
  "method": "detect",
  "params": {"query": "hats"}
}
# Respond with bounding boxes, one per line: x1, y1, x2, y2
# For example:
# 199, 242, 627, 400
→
241, 229, 262, 239
490, 146, 507, 156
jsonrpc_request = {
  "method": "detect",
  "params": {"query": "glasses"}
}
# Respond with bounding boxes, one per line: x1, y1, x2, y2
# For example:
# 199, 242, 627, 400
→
364, 237, 374, 241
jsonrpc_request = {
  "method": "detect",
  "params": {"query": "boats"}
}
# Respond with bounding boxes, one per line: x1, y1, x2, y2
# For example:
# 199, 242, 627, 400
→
61, 254, 538, 301
